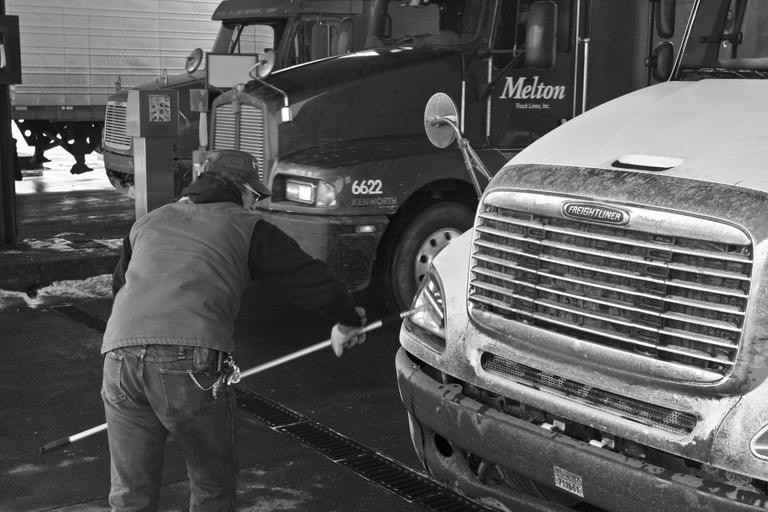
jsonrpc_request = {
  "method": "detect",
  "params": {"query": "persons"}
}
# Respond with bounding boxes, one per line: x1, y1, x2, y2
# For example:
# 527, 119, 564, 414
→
100, 149, 366, 511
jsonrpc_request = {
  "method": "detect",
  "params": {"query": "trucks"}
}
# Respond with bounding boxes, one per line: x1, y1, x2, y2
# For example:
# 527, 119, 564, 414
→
95, 1, 437, 203
187, 0, 768, 339
0, 0, 304, 175
385, 0, 768, 512
0, 0, 24, 249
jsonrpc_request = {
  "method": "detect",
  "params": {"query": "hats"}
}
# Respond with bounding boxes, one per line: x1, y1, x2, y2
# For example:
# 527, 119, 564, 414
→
200, 148, 273, 200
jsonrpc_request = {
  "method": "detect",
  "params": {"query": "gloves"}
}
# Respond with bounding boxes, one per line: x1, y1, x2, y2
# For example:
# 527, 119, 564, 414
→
330, 305, 367, 359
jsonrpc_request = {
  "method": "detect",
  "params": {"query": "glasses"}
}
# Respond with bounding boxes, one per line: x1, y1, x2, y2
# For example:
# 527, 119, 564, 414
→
241, 181, 262, 202
